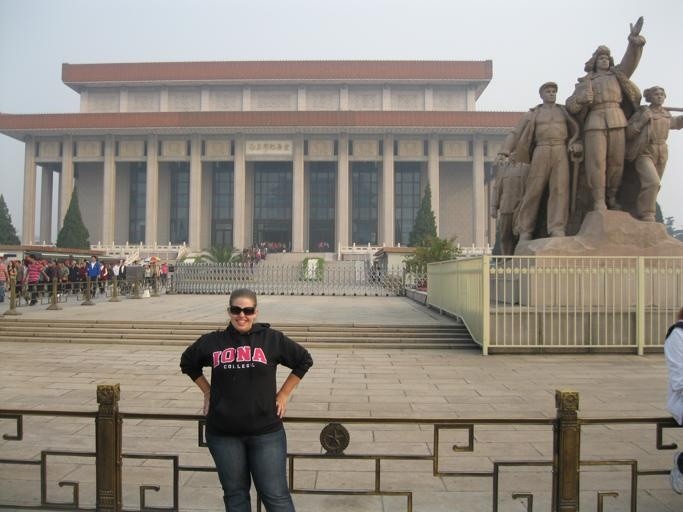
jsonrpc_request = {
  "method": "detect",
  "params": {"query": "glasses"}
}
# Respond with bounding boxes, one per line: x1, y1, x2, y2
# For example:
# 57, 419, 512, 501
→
230, 305, 255, 315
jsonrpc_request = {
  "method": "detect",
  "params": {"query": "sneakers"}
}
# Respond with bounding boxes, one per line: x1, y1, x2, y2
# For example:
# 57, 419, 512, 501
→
669, 451, 683, 495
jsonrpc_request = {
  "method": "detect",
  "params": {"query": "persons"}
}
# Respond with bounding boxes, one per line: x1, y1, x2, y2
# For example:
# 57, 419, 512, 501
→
563, 14, 648, 210
0, 252, 173, 306
176, 287, 315, 512
487, 149, 531, 264
322, 240, 329, 252
233, 237, 291, 275
493, 80, 583, 243
317, 241, 324, 251
624, 84, 682, 224
659, 306, 683, 498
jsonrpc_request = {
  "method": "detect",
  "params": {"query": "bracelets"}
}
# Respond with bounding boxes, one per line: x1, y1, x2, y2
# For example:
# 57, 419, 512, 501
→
203, 390, 210, 397
